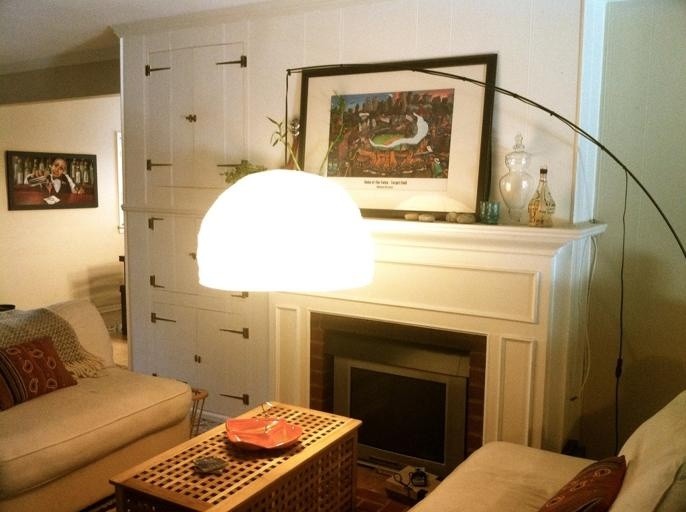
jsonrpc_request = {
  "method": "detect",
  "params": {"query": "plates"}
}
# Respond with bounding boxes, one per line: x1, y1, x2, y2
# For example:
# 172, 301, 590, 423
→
221, 417, 303, 450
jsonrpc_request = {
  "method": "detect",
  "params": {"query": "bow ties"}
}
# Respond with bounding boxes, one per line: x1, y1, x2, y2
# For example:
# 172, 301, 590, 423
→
52, 176, 61, 180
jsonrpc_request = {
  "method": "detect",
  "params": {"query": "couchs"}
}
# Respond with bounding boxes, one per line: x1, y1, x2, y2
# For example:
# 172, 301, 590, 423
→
0, 296, 196, 512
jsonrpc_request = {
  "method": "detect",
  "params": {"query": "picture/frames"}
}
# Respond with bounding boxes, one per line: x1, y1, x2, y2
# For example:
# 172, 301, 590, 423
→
3, 149, 99, 212
296, 52, 499, 227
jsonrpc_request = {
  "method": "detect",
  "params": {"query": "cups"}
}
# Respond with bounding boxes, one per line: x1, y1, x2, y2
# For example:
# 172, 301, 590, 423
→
479, 197, 501, 225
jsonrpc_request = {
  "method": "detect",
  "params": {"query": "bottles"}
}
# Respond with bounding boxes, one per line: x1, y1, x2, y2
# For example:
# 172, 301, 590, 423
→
525, 164, 556, 227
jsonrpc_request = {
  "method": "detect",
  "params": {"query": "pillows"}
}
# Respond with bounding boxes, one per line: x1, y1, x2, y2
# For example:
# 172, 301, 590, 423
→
539, 455, 627, 512
0, 332, 79, 416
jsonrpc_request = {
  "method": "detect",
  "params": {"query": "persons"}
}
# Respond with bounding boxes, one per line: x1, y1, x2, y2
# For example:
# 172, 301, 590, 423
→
27, 155, 86, 202
10, 153, 95, 186
329, 92, 453, 182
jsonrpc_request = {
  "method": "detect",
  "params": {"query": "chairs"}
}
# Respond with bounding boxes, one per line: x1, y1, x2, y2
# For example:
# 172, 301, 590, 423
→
405, 389, 685, 512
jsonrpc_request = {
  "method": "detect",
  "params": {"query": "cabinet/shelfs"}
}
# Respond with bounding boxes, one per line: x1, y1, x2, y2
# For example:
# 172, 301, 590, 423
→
118, 15, 272, 425
